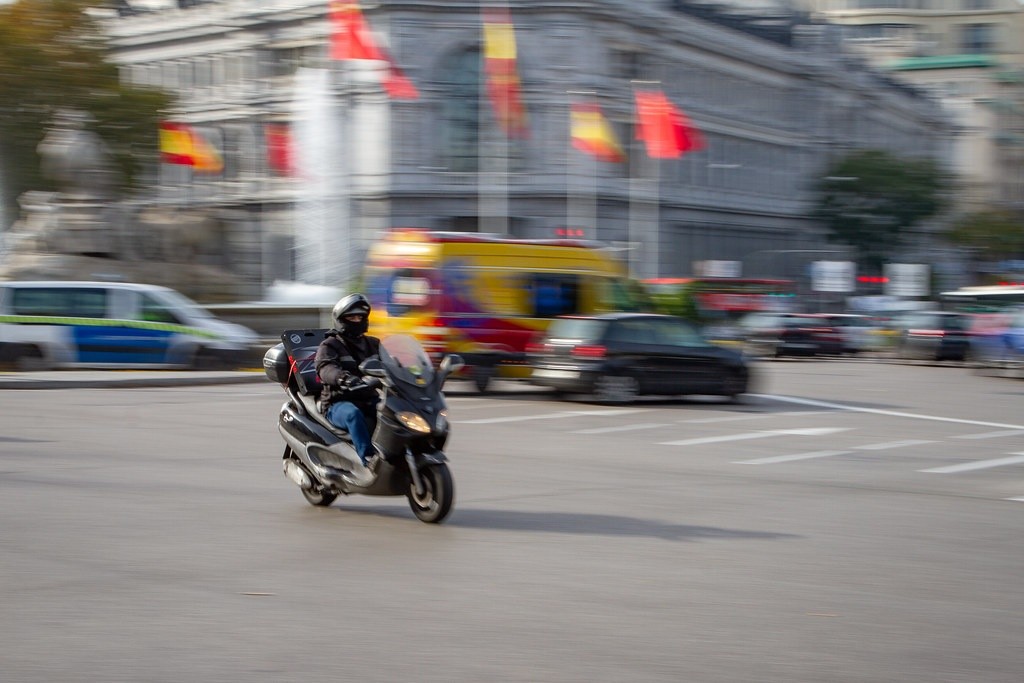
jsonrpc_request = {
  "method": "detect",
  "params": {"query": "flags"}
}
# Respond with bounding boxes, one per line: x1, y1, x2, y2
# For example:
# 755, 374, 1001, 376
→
569, 102, 628, 163
263, 120, 294, 175
481, 0, 531, 139
159, 119, 224, 175
634, 88, 707, 159
325, 0, 419, 98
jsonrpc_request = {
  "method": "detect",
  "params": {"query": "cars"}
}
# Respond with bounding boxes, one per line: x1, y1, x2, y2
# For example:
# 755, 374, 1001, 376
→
522, 309, 749, 408
640, 269, 1024, 366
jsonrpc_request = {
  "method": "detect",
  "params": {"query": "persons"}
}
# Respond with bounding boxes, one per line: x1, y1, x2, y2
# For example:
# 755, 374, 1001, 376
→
316, 292, 396, 473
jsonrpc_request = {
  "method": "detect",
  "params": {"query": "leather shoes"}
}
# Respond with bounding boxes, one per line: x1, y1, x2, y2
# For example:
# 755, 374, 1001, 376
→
365, 454, 381, 471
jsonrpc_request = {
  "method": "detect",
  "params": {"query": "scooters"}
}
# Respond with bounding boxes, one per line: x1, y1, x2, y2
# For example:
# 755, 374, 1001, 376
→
262, 331, 465, 525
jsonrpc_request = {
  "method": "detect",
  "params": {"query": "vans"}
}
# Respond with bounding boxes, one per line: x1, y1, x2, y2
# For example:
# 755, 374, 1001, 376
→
0, 280, 262, 372
360, 226, 660, 390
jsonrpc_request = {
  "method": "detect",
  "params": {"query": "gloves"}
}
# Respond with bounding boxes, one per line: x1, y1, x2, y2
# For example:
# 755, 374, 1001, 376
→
344, 375, 363, 386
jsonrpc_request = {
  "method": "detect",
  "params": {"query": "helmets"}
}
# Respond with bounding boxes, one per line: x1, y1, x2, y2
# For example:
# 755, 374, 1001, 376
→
332, 293, 371, 335
